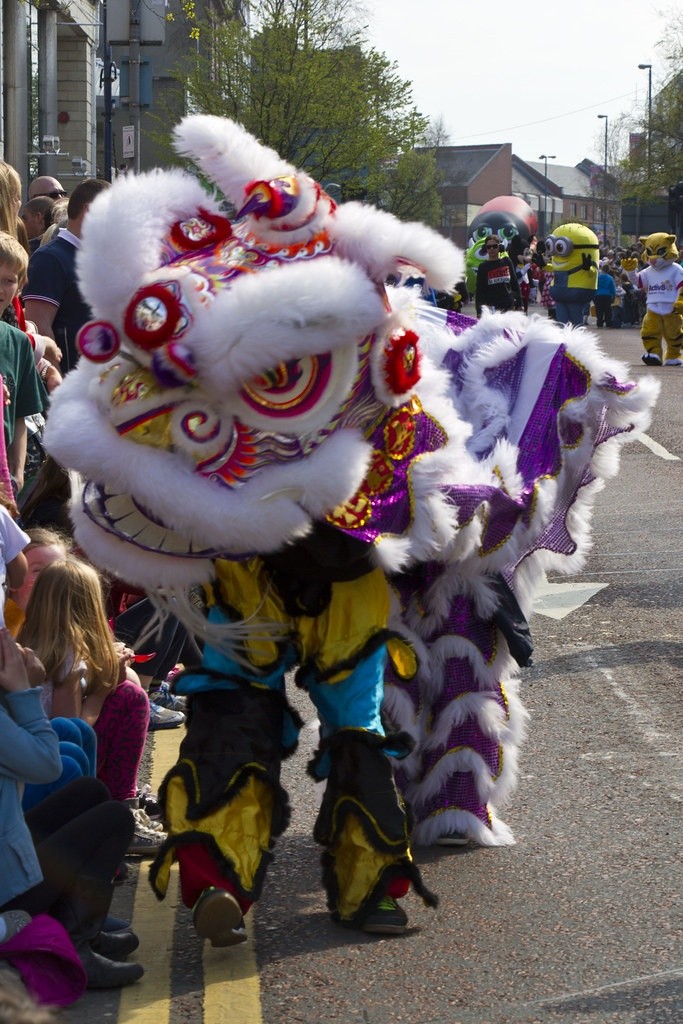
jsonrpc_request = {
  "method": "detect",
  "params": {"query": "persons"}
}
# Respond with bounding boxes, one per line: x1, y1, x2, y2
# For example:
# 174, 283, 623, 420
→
474, 233, 683, 330
1, 162, 191, 1024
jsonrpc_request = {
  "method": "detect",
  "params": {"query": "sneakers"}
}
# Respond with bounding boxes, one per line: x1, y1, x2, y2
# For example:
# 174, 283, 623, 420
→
124, 783, 168, 854
192, 885, 247, 949
146, 681, 187, 730
359, 895, 409, 933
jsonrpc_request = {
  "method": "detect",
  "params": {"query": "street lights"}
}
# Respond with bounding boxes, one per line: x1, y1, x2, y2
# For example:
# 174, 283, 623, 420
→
638, 61, 660, 232
597, 114, 608, 245
539, 155, 556, 234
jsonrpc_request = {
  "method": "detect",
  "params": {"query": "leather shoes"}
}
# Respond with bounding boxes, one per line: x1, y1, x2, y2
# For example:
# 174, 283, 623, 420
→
88, 930, 145, 960
77, 941, 144, 991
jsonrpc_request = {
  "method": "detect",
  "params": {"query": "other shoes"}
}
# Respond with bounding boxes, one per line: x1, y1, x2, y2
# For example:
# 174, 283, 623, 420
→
101, 914, 131, 933
439, 833, 469, 846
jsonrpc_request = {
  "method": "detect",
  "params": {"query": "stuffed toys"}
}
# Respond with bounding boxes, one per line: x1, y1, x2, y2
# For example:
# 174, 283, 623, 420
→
621, 232, 683, 366
45, 114, 660, 946
465, 196, 538, 300
545, 222, 600, 326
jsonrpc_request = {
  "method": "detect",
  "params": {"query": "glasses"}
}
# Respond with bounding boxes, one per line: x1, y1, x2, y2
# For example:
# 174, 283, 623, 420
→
34, 192, 67, 199
487, 244, 498, 249
